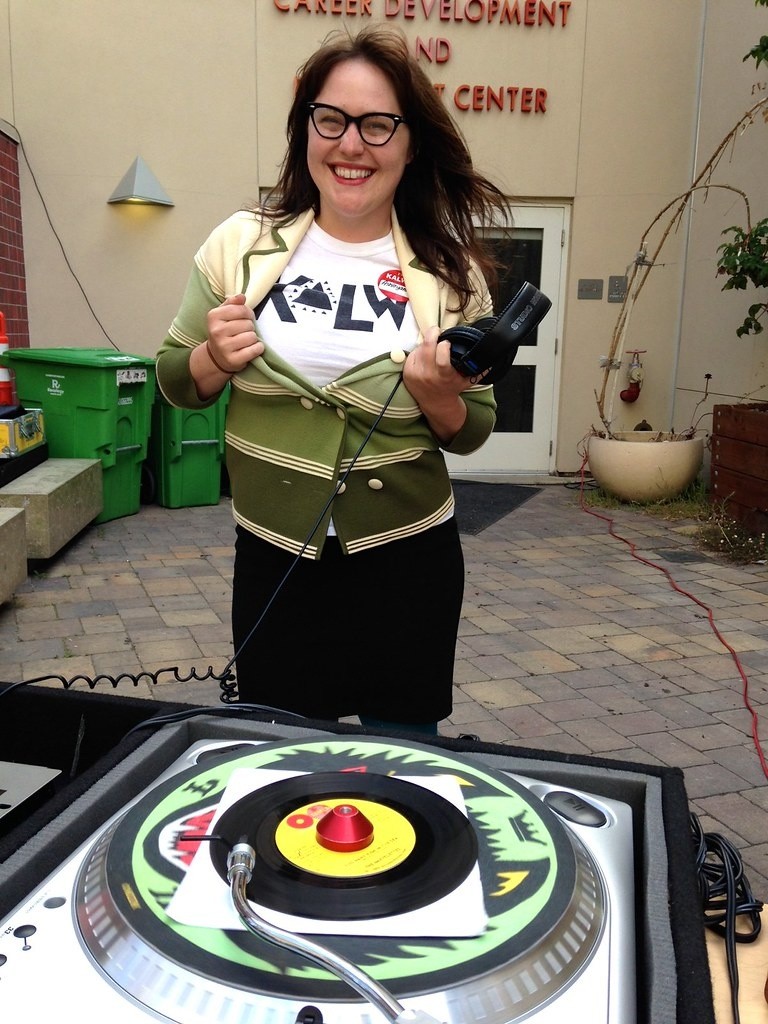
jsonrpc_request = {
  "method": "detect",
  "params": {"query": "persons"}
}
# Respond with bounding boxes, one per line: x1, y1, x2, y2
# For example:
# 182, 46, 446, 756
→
155, 18, 519, 737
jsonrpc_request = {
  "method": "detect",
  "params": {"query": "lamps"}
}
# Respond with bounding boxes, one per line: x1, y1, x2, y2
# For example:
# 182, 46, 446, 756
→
107, 153, 175, 207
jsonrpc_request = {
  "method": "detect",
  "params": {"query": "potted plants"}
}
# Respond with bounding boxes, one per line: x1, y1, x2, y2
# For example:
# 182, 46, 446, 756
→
705, 217, 768, 540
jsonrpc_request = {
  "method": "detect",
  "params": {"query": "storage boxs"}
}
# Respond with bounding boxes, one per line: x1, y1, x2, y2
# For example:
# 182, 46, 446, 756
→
0, 408, 47, 458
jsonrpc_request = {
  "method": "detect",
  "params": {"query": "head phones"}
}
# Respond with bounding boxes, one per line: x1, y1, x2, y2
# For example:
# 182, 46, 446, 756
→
436, 280, 552, 385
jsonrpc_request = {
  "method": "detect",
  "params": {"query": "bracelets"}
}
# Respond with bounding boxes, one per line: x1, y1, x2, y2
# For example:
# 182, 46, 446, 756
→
205, 341, 249, 374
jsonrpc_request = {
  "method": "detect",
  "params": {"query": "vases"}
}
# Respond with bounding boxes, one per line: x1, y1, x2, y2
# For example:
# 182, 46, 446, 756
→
586, 428, 704, 505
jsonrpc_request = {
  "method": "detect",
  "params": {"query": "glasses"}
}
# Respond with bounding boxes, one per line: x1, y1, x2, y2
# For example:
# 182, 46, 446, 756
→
306, 103, 405, 146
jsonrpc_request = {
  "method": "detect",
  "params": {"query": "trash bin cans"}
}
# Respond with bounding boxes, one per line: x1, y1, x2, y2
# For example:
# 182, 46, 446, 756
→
140, 358, 231, 508
0, 348, 157, 524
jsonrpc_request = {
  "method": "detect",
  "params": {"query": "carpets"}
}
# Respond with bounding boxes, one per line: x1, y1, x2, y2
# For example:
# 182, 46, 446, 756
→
450, 479, 544, 537
